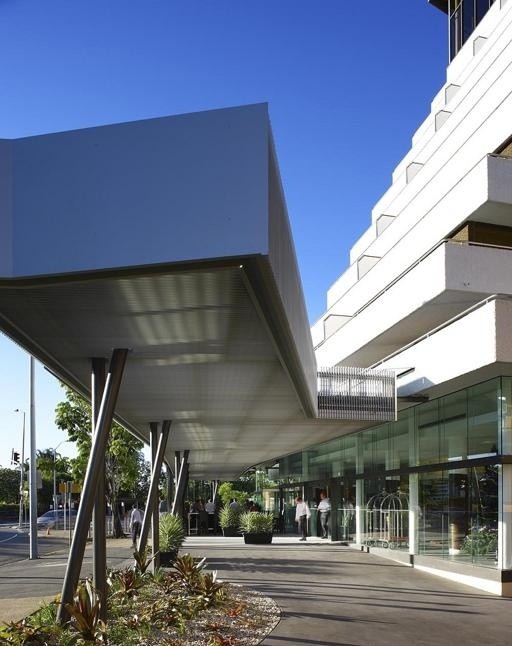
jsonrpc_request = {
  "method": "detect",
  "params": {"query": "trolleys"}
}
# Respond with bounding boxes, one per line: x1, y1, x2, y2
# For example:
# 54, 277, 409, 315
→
380, 486, 422, 550
363, 486, 391, 548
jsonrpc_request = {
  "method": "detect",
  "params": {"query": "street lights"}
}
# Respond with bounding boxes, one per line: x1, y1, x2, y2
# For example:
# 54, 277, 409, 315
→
51, 439, 70, 530
15, 407, 26, 528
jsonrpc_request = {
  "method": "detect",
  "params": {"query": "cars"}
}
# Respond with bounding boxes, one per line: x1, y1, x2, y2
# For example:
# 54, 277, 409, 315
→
37, 509, 80, 530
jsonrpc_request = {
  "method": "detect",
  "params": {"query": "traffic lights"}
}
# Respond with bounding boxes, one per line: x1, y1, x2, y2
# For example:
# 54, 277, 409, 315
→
14, 452, 20, 462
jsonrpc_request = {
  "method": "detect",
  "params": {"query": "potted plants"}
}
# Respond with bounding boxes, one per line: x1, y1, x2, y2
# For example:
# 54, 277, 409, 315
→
159, 513, 186, 567
217, 505, 275, 545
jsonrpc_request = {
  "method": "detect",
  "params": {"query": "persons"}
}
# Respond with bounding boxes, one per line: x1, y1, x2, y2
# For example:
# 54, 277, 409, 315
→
316, 492, 332, 540
158, 495, 259, 536
294, 497, 311, 541
129, 504, 144, 547
